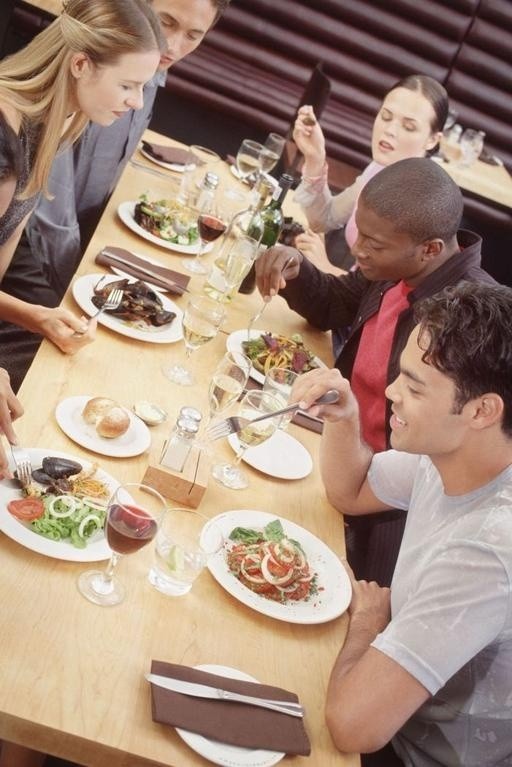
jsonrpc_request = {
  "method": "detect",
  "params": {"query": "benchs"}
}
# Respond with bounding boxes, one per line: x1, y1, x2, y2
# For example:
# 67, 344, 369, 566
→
0, 1, 511, 235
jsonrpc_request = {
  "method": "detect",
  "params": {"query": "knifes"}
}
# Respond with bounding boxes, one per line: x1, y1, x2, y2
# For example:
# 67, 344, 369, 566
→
142, 670, 304, 718
101, 248, 191, 293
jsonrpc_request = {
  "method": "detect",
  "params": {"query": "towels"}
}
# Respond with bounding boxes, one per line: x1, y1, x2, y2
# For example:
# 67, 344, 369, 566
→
94, 246, 192, 297
148, 657, 311, 760
141, 140, 207, 167
225, 152, 276, 197
237, 374, 326, 439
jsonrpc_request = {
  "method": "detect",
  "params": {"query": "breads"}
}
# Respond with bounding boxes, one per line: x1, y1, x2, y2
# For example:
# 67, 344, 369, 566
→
84, 397, 130, 438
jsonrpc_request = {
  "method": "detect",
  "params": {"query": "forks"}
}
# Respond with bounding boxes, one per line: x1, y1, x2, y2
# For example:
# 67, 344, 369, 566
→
246, 257, 294, 346
204, 389, 339, 442
75, 287, 126, 336
9, 441, 31, 481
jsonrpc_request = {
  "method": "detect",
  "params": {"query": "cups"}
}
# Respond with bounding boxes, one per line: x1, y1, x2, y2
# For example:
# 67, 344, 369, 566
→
176, 144, 218, 202
439, 110, 484, 167
149, 509, 224, 598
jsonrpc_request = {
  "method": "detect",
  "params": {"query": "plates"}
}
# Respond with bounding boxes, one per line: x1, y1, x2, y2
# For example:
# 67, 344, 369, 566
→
55, 395, 151, 459
229, 431, 314, 480
203, 508, 352, 625
0, 449, 137, 564
178, 665, 288, 765
118, 200, 213, 254
142, 144, 197, 173
74, 271, 187, 341
228, 327, 332, 391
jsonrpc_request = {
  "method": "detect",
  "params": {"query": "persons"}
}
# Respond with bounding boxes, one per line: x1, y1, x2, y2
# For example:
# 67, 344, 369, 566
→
1, 3, 168, 482
291, 71, 451, 277
6, 1, 230, 253
1, 106, 27, 450
276, 276, 512, 767
252, 154, 503, 588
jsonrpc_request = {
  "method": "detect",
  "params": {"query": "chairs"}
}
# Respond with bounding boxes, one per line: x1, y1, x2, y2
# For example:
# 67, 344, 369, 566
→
451, 183, 509, 284
271, 59, 369, 194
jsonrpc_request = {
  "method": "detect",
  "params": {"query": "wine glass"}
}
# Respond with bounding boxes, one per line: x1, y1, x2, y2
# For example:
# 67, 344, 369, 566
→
167, 293, 225, 384
184, 197, 231, 272
207, 350, 307, 489
226, 132, 287, 202
78, 483, 170, 606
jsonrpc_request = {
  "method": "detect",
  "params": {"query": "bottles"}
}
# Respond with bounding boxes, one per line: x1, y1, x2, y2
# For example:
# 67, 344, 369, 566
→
195, 172, 220, 208
164, 407, 203, 466
237, 173, 295, 294
206, 179, 273, 299
